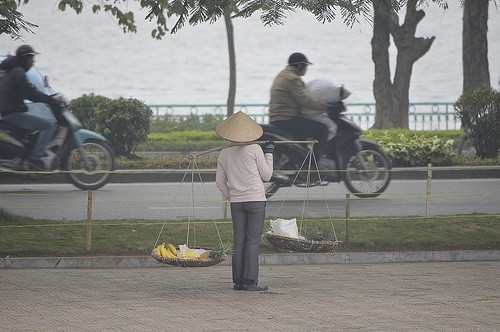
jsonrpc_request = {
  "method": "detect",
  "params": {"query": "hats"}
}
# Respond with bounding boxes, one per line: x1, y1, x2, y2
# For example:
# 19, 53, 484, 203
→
288, 52, 312, 65
17, 45, 39, 57
216, 110, 263, 142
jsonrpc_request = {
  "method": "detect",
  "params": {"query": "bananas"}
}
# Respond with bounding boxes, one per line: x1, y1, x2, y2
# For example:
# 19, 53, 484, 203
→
157, 242, 177, 258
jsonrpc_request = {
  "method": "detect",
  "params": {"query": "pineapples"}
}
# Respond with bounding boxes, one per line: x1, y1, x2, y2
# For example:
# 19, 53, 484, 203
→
198, 243, 235, 259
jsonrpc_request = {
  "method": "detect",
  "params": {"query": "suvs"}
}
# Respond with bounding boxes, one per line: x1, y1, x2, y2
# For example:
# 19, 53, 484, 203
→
0, 53, 58, 122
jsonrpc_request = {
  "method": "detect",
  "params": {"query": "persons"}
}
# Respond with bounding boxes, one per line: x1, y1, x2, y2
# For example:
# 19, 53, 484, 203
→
0, 44, 68, 172
268, 53, 330, 187
215, 111, 275, 291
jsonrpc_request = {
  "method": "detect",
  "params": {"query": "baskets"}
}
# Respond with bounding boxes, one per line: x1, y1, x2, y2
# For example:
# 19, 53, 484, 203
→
266, 233, 338, 253
150, 244, 226, 267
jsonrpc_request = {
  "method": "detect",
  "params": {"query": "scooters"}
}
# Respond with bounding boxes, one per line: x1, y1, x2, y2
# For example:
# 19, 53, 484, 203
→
256, 85, 392, 199
1, 92, 113, 189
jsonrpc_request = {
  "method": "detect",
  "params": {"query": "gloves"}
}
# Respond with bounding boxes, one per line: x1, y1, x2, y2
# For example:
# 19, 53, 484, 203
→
263, 139, 275, 154
331, 102, 345, 113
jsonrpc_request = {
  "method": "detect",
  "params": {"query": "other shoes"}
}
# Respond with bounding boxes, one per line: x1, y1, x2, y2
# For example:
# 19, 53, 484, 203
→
19, 159, 47, 171
241, 283, 268, 291
233, 282, 243, 289
304, 164, 329, 175
